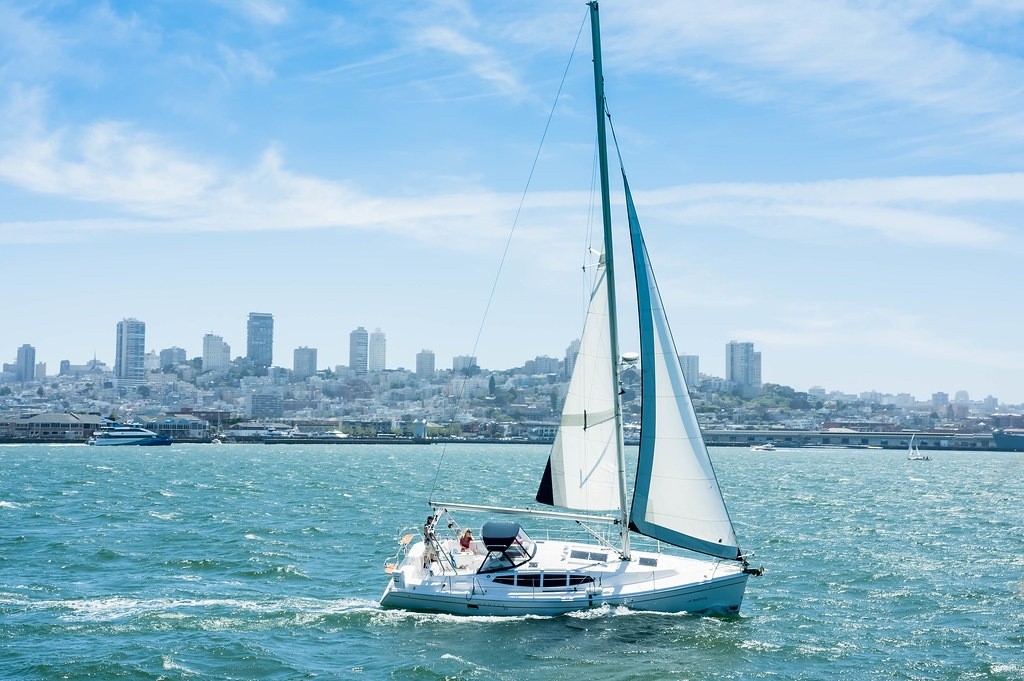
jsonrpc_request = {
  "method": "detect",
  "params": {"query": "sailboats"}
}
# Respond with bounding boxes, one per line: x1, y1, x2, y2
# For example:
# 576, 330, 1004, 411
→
907, 433, 927, 460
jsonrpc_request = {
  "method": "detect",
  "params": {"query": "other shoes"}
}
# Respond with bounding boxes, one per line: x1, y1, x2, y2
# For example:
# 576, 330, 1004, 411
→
431, 559, 437, 562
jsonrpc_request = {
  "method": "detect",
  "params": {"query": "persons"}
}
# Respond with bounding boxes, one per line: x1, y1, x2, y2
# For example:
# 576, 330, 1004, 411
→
460, 530, 474, 552
424, 517, 437, 569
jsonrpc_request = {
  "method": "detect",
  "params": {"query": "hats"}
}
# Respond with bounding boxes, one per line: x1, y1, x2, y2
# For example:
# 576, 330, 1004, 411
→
428, 517, 434, 519
467, 530, 472, 533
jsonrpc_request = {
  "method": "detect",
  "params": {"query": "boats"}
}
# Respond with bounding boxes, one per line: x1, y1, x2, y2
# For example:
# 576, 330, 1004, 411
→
995, 429, 1024, 452
750, 442, 777, 450
88, 415, 174, 447
379, 0, 765, 614
